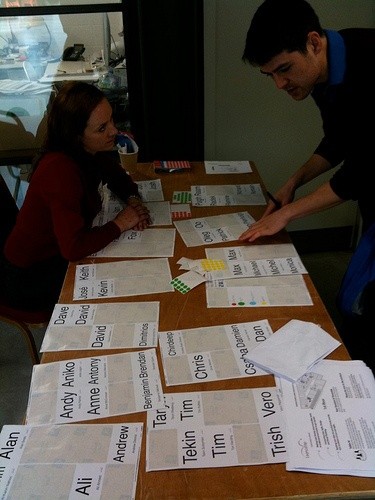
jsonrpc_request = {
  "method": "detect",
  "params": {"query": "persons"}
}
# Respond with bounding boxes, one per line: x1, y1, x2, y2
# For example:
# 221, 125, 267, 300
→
239, 0, 375, 373
0, 80, 153, 310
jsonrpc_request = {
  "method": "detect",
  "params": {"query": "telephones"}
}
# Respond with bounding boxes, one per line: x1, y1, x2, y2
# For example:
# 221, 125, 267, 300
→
62, 43, 86, 61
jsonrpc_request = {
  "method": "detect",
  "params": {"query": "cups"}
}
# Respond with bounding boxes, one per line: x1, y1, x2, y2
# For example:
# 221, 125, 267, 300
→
118, 147, 139, 175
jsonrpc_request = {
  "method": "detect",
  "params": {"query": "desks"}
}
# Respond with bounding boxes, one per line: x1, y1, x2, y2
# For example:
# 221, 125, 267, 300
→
0, 50, 126, 169
25, 161, 375, 500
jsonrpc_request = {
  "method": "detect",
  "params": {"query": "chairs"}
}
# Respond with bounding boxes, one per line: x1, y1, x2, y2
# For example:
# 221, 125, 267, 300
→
0, 174, 52, 365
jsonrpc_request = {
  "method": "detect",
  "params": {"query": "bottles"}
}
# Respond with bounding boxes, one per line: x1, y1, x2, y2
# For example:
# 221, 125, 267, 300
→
100, 75, 113, 89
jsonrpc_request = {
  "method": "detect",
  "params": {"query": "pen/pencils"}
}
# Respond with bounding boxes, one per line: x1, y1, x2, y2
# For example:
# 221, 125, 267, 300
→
265, 190, 281, 207
117, 142, 135, 153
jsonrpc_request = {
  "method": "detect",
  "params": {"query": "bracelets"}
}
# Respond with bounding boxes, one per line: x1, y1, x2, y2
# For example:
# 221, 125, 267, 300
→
127, 195, 141, 201
